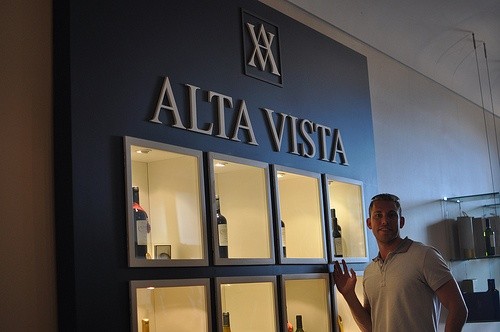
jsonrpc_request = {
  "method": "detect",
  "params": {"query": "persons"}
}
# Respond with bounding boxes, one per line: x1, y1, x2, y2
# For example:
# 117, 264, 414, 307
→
333, 194, 468, 332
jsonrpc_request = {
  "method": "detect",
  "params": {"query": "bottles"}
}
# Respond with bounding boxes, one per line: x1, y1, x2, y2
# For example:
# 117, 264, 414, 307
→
331, 209, 336, 219
222, 312, 231, 332
332, 218, 343, 257
482, 218, 496, 257
295, 314, 304, 332
281, 220, 287, 258
133, 186, 148, 259
141, 319, 150, 332
215, 195, 229, 258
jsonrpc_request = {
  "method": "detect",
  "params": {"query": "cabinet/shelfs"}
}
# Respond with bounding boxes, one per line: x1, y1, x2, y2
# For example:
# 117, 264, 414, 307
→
122, 134, 370, 331
439, 191, 500, 323
